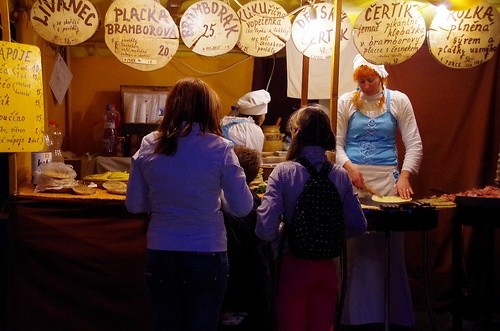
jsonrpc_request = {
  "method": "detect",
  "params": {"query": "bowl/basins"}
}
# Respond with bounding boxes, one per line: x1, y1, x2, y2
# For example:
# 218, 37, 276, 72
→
263, 132, 285, 152
261, 125, 280, 132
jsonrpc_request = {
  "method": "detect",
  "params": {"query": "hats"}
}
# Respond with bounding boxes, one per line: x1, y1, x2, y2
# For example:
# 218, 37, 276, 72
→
353, 53, 389, 78
237, 89, 271, 115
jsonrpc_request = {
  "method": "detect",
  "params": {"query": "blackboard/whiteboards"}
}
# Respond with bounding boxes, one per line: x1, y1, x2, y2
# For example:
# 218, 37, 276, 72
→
0, 41, 45, 152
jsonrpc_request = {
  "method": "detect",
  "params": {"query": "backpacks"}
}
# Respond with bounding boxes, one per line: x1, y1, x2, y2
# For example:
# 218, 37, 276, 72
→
288, 157, 345, 260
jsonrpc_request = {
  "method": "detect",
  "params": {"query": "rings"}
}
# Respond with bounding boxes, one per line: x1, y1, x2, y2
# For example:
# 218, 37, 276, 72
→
407, 188, 409, 192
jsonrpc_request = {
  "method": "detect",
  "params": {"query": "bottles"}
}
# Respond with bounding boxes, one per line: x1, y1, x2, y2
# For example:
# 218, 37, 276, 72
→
102, 103, 140, 157
48, 121, 64, 161
31, 128, 53, 185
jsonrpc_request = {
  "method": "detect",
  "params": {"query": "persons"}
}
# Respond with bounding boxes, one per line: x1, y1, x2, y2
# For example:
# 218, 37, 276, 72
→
335, 54, 423, 331
255, 106, 367, 331
220, 144, 277, 331
125, 77, 253, 331
218, 88, 271, 191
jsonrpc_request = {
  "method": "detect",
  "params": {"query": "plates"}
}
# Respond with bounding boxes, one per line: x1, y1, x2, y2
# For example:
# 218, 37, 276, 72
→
84, 175, 130, 181
370, 195, 412, 205
